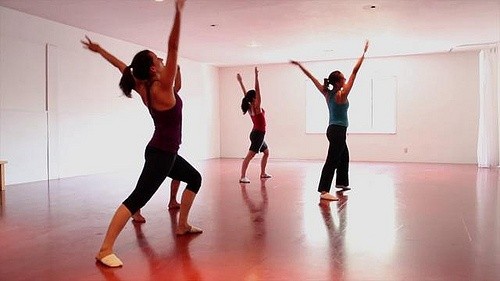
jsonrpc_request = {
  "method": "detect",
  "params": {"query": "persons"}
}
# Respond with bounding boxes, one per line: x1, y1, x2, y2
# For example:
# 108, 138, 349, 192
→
81, 0, 203, 267
289, 40, 369, 201
236, 67, 272, 183
129, 64, 182, 222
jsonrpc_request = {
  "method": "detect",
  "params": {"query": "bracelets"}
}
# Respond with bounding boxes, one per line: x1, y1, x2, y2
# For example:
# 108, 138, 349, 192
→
363, 51, 366, 55
